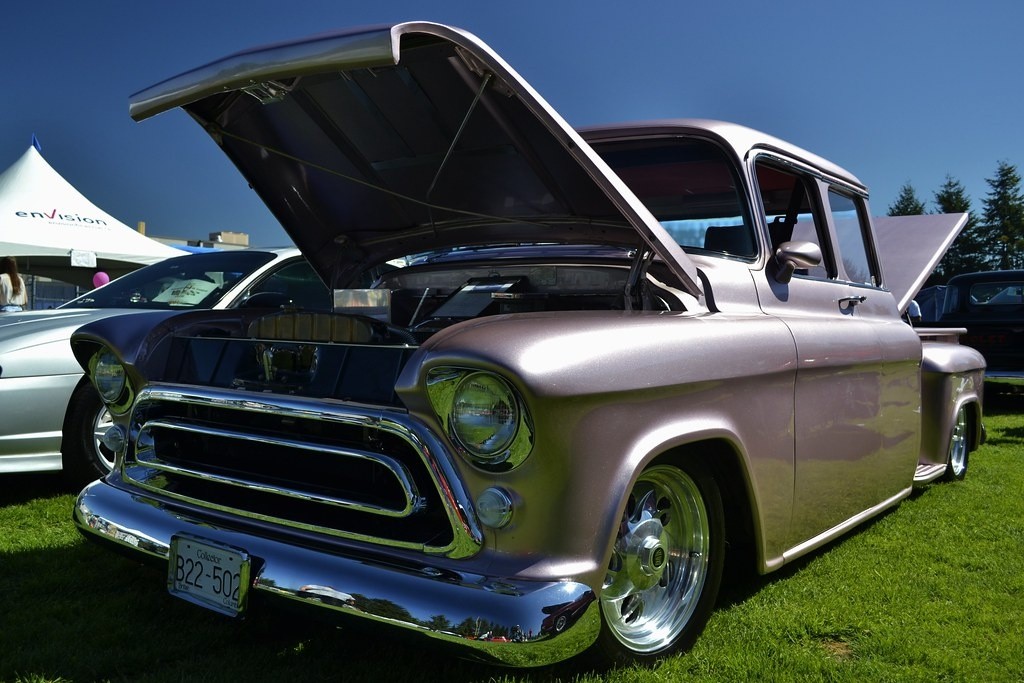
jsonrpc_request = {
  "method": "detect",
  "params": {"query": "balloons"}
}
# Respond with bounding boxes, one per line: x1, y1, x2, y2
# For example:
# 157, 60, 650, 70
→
93, 271, 110, 287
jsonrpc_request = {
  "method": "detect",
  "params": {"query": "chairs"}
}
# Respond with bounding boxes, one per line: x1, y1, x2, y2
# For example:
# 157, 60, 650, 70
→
703, 224, 748, 253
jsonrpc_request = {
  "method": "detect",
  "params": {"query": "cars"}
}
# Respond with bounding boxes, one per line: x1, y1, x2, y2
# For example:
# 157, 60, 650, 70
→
911, 269, 1024, 395
72, 18, 987, 670
0, 246, 406, 492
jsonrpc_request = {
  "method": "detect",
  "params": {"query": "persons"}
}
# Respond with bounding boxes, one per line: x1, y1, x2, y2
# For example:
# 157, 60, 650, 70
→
908, 300, 922, 322
0, 256, 28, 312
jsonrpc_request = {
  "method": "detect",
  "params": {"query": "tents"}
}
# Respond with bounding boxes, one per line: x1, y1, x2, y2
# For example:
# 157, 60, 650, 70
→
0, 145, 193, 291
